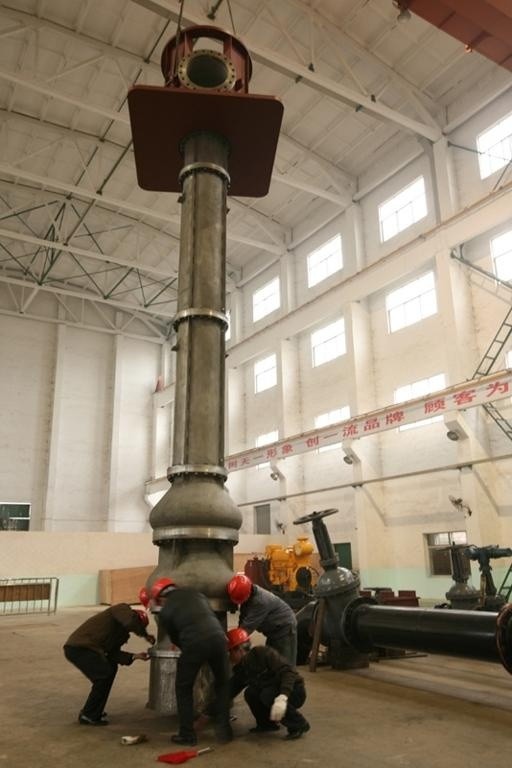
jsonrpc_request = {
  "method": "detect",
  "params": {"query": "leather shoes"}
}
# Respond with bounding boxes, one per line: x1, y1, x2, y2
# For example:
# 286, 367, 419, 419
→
287, 723, 310, 739
80, 715, 108, 726
250, 725, 280, 732
171, 735, 197, 746
217, 729, 232, 741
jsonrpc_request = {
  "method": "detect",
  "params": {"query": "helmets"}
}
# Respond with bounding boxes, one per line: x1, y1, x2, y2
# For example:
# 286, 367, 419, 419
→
151, 578, 175, 599
139, 588, 149, 609
133, 609, 149, 628
226, 628, 249, 650
227, 575, 252, 605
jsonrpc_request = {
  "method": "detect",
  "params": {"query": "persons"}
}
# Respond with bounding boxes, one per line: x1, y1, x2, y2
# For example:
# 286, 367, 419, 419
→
151, 576, 234, 746
61, 601, 157, 728
226, 573, 300, 673
191, 627, 312, 741
138, 584, 154, 611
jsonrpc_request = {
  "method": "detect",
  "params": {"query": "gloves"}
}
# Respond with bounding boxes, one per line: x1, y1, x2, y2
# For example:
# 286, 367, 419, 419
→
121, 734, 148, 745
269, 694, 288, 721
